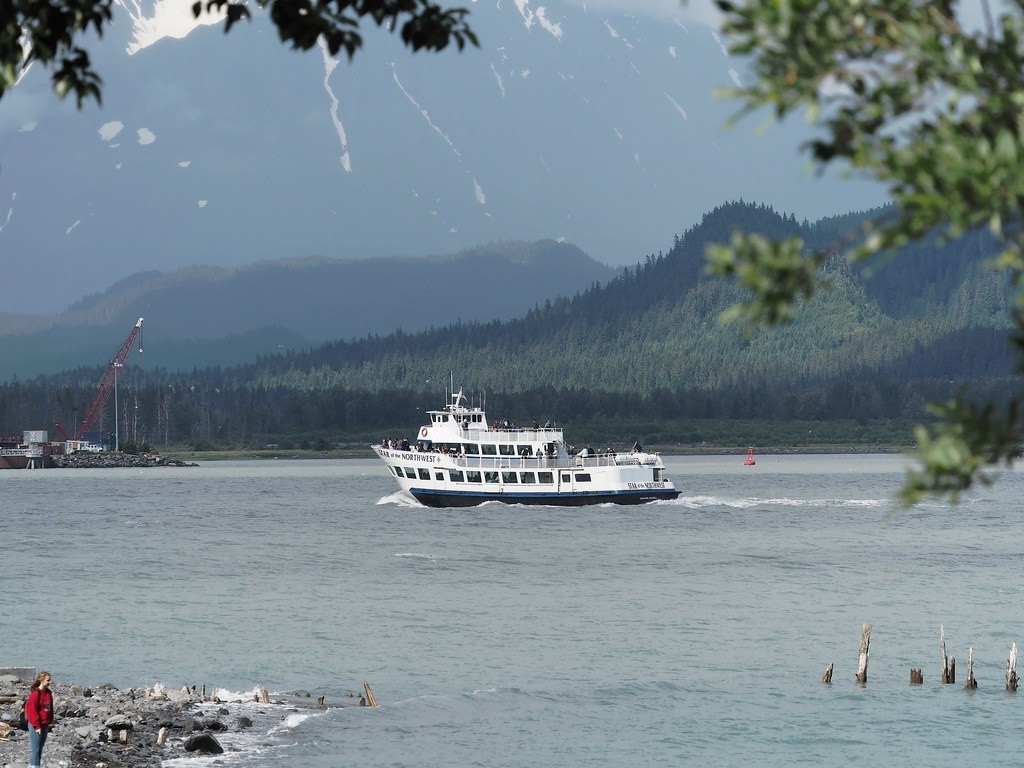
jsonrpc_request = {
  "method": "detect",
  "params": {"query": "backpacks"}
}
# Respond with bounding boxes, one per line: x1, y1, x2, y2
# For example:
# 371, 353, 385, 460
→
19, 690, 41, 731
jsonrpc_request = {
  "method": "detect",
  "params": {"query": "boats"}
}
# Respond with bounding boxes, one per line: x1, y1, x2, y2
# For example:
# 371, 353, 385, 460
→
371, 370, 685, 507
744, 448, 756, 465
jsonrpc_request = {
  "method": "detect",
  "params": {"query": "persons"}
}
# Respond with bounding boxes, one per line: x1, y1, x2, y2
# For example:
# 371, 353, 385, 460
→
493, 418, 517, 432
545, 420, 551, 431
521, 447, 529, 459
504, 449, 514, 455
533, 417, 537, 432
419, 443, 431, 452
383, 437, 410, 451
536, 448, 543, 459
25, 672, 54, 768
545, 446, 616, 459
441, 447, 448, 453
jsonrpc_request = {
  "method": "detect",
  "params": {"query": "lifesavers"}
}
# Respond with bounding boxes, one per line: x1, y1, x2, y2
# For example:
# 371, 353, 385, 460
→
421, 428, 428, 437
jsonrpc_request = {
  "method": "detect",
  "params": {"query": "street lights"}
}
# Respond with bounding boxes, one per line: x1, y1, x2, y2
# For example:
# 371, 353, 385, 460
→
111, 357, 124, 450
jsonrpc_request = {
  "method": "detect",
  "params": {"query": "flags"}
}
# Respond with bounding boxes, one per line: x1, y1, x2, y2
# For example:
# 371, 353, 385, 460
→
635, 443, 642, 452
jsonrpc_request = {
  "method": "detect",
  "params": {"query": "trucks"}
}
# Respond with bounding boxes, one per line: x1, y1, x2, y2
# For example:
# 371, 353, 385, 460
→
88, 445, 103, 453
